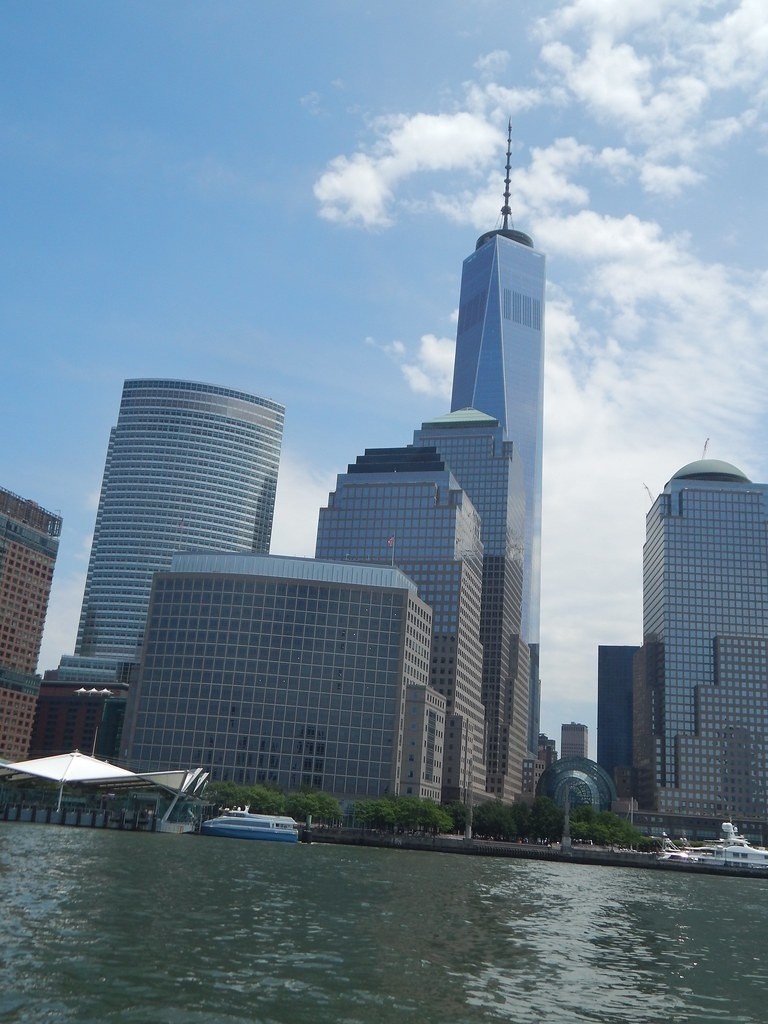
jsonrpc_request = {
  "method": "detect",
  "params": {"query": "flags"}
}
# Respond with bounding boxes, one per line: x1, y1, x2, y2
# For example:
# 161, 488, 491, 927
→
388, 535, 394, 547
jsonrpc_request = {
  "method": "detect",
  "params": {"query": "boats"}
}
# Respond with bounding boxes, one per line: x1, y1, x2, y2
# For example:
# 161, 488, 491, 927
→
656, 816, 768, 877
202, 805, 298, 843
